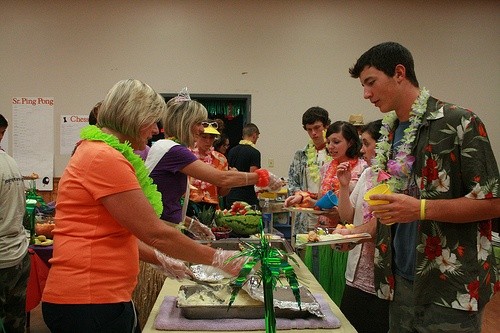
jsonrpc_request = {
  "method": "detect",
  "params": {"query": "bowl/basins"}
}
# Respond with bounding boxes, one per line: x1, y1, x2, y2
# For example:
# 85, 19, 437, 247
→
211, 226, 232, 240
35, 215, 55, 240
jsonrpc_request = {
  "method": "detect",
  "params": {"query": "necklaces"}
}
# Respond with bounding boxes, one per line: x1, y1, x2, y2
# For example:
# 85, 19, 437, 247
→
362, 168, 375, 255
80, 125, 164, 218
306, 129, 333, 183
191, 145, 215, 166
371, 87, 433, 193
321, 157, 360, 197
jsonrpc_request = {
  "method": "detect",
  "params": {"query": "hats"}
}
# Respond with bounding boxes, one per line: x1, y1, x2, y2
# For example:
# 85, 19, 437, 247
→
203, 125, 220, 134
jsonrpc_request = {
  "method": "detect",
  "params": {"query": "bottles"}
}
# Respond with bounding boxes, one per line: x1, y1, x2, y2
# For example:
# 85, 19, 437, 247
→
262, 198, 273, 234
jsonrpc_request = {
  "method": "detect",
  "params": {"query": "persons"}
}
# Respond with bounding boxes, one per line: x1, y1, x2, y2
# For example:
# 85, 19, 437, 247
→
145, 88, 284, 242
286, 122, 368, 311
0, 113, 31, 333
183, 119, 239, 226
337, 119, 389, 333
349, 42, 500, 333
213, 122, 283, 211
41, 79, 255, 333
286, 106, 334, 262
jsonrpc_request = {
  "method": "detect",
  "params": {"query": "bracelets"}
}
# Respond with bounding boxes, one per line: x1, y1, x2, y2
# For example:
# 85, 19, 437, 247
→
245, 173, 249, 186
420, 199, 426, 220
255, 168, 270, 188
187, 218, 194, 230
299, 194, 304, 204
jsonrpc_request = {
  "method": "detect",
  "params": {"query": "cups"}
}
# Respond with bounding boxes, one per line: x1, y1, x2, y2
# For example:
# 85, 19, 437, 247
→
363, 184, 395, 225
314, 190, 339, 211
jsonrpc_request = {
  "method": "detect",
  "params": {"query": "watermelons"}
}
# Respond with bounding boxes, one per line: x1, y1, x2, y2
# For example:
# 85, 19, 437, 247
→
215, 214, 262, 237
230, 201, 251, 214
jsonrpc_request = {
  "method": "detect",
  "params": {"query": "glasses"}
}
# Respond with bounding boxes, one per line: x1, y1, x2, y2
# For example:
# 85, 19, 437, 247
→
223, 144, 230, 148
202, 122, 218, 129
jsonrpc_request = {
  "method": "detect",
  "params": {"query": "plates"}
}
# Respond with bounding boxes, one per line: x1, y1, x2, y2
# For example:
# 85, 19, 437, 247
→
295, 232, 372, 248
282, 207, 330, 214
193, 272, 239, 286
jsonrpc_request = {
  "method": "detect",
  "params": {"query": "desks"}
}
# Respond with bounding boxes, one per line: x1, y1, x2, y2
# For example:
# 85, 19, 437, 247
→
142, 251, 357, 333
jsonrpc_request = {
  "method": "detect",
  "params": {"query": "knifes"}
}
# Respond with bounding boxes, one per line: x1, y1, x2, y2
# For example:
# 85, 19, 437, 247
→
186, 273, 213, 288
